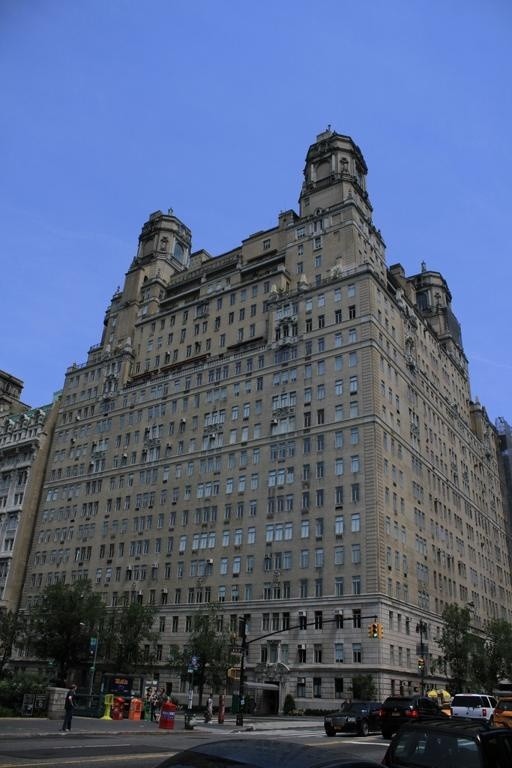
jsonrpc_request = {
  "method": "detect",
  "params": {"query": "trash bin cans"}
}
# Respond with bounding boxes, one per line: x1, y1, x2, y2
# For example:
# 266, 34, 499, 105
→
159, 702, 177, 729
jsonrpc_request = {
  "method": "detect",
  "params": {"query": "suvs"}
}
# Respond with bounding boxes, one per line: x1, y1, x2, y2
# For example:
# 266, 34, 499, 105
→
450, 690, 502, 729
321, 698, 384, 740
380, 714, 512, 768
486, 696, 512, 731
375, 692, 453, 744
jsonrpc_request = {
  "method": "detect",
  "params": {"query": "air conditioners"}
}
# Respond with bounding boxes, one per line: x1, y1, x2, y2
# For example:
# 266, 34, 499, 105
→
126, 559, 214, 597
333, 608, 343, 615
70, 416, 278, 465
298, 610, 307, 617
297, 678, 305, 684
298, 644, 305, 650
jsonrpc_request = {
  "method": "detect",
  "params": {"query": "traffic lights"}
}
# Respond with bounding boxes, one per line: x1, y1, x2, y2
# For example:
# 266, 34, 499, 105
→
418, 658, 426, 672
378, 623, 386, 640
367, 623, 373, 639
371, 623, 379, 639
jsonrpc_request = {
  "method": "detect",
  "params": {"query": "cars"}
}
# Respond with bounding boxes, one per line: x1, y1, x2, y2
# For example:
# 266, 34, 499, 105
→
147, 735, 388, 767
440, 700, 454, 717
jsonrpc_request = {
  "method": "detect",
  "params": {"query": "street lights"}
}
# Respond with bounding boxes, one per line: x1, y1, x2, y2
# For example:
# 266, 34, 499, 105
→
414, 615, 430, 696
80, 620, 102, 710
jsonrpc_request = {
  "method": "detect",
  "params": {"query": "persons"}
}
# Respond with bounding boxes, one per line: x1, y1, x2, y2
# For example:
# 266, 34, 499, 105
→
340, 699, 348, 708
150, 687, 169, 723
62, 684, 79, 732
207, 693, 213, 724
218, 694, 226, 724
